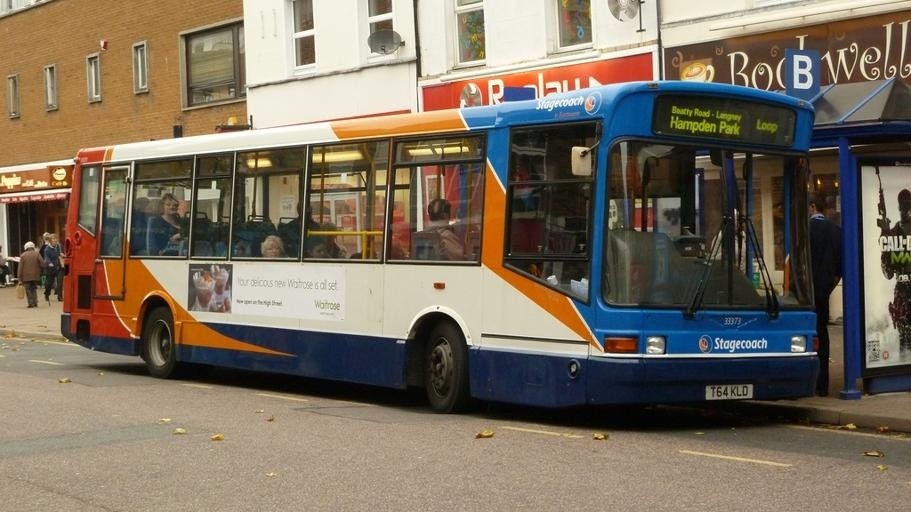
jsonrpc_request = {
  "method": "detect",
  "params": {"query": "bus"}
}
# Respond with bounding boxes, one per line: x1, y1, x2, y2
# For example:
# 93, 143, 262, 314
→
58, 80, 824, 422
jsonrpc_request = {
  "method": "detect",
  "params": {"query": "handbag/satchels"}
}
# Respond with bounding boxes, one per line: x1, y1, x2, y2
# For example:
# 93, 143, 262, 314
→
15, 285, 25, 299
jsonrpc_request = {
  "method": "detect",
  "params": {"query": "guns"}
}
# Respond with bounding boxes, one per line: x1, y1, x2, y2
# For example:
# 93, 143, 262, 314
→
876, 165, 904, 278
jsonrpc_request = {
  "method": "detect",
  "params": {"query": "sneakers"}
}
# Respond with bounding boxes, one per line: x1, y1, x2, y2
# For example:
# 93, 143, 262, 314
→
46, 293, 64, 301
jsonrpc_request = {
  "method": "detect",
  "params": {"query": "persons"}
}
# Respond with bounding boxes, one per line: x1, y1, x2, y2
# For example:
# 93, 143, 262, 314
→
94, 193, 592, 285
0, 227, 74, 308
753, 191, 789, 271
710, 208, 749, 278
804, 189, 845, 397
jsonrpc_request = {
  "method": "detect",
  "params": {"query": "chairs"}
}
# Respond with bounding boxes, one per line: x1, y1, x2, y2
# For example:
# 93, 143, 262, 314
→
409, 232, 444, 261
145, 211, 298, 257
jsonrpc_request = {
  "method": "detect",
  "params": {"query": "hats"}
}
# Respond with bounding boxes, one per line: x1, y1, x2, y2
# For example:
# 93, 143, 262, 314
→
898, 189, 910, 210
44, 231, 50, 238
24, 241, 34, 250
